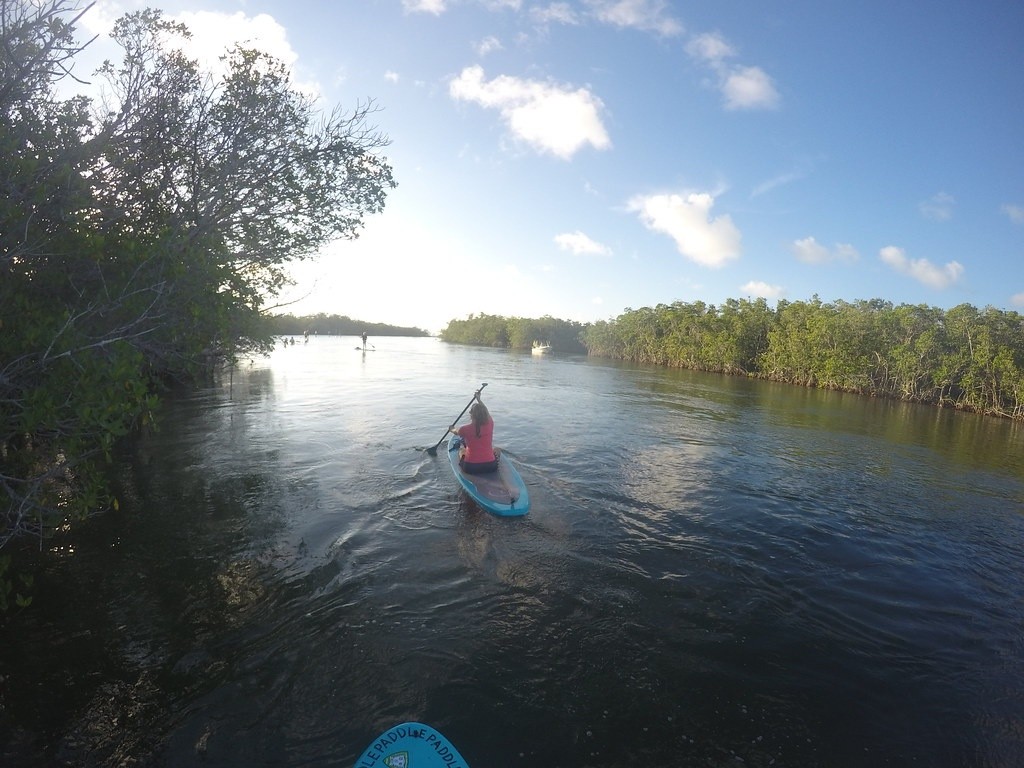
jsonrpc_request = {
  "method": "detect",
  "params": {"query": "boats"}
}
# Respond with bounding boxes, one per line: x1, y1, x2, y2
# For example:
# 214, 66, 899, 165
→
531, 335, 552, 356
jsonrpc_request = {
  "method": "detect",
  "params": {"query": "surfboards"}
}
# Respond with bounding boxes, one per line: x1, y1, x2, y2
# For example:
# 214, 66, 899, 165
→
447, 432, 529, 517
353, 722, 468, 768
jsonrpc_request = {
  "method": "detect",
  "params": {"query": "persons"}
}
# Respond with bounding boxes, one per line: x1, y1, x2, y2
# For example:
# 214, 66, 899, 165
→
314, 328, 318, 338
360, 332, 367, 349
328, 330, 331, 338
303, 329, 310, 340
448, 389, 501, 474
283, 337, 295, 345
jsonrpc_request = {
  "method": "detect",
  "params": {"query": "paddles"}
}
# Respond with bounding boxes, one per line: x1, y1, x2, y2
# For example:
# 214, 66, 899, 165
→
427, 380, 489, 455
